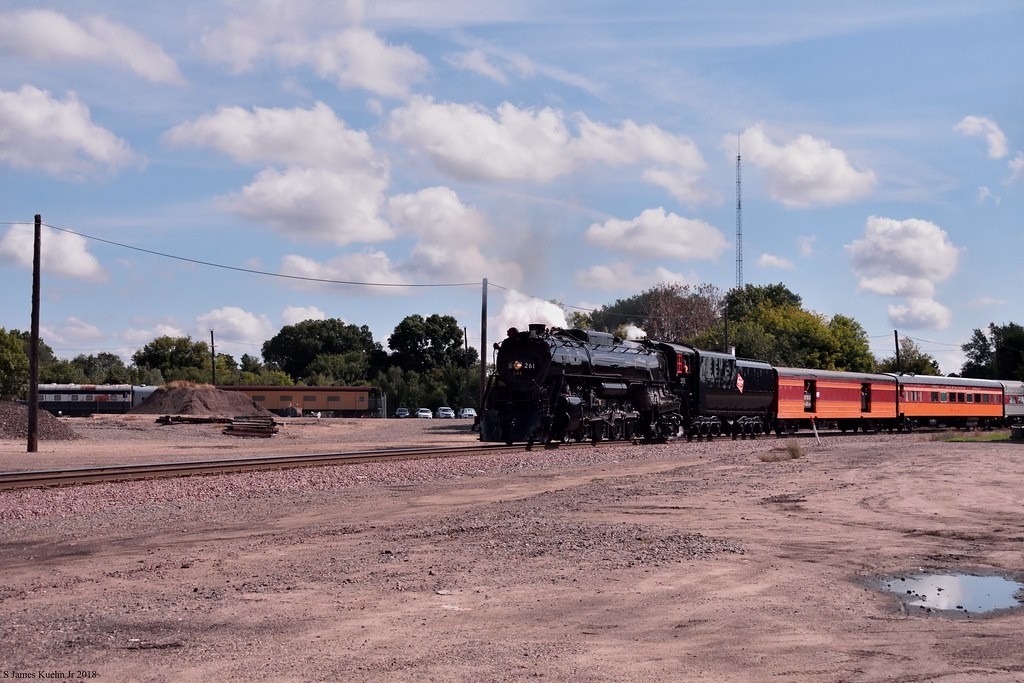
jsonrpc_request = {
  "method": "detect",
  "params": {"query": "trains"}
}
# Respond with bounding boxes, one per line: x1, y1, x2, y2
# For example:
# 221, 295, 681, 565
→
18, 383, 384, 418
472, 322, 1024, 447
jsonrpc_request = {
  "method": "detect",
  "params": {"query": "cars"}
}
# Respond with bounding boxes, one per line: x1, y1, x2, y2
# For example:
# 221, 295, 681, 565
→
395, 408, 410, 418
437, 407, 455, 419
415, 408, 433, 419
457, 409, 477, 419
